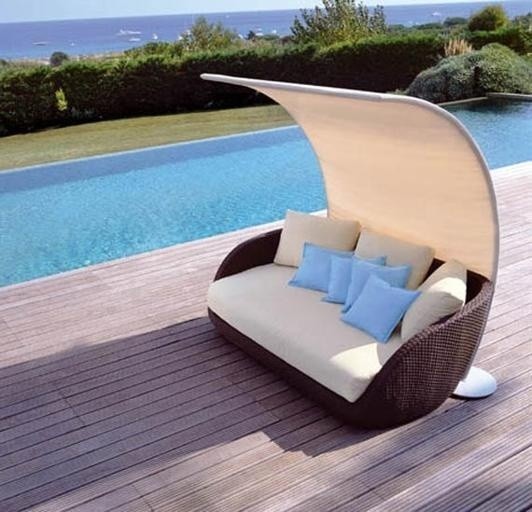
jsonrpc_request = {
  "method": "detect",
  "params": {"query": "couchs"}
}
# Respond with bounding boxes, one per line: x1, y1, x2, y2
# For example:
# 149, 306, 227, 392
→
203, 228, 496, 429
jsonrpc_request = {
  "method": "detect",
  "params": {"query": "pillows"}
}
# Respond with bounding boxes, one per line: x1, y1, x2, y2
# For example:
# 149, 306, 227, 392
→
269, 208, 468, 345
